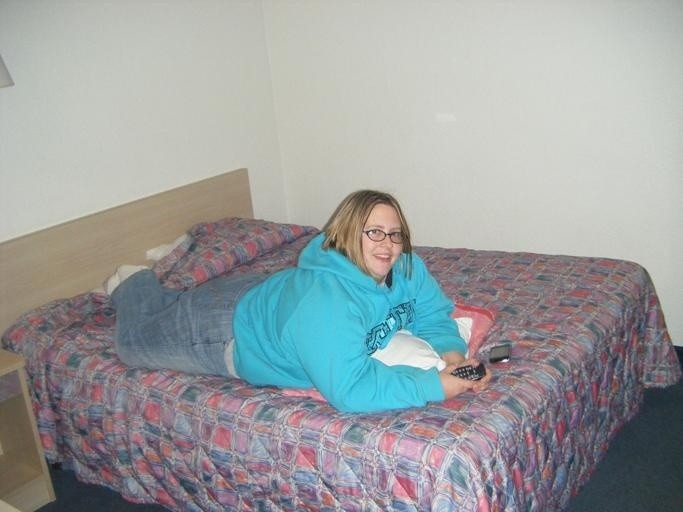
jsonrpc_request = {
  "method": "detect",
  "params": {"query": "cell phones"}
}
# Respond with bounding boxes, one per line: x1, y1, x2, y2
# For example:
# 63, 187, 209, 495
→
490, 344, 510, 363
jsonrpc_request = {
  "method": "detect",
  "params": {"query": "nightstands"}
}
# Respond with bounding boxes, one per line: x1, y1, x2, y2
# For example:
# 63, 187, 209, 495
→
0, 349, 56, 511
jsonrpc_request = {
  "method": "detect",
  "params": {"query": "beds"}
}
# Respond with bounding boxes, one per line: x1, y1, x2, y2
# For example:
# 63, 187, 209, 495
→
0, 169, 650, 511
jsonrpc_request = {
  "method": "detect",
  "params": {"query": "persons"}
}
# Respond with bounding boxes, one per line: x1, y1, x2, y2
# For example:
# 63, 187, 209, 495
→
106, 189, 492, 414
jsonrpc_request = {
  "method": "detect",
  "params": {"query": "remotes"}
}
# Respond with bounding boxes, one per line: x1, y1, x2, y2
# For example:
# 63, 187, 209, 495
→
451, 364, 486, 381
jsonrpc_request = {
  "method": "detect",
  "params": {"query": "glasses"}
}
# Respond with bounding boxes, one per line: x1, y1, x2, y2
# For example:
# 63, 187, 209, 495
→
361, 229, 406, 244
